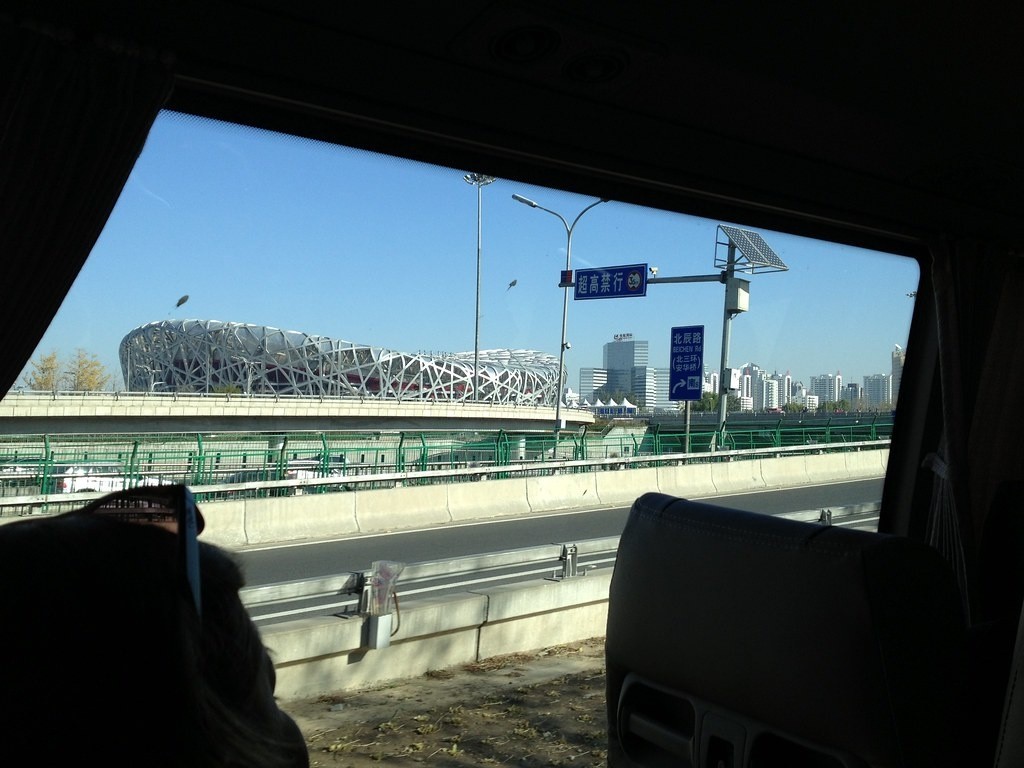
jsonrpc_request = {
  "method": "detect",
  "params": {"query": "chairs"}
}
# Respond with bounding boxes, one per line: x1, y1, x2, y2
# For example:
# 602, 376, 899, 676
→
605, 492, 1023, 768
979, 480, 1023, 557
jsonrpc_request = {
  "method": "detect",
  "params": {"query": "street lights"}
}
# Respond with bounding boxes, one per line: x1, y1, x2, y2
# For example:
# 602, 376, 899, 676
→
513, 193, 611, 459
463, 174, 500, 400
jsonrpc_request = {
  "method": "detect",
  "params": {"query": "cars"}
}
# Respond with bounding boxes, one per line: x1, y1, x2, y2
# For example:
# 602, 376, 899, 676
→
218, 460, 341, 499
310, 455, 351, 470
57, 459, 177, 494
0, 457, 56, 486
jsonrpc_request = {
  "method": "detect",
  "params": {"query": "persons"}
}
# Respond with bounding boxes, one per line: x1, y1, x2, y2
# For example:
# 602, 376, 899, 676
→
1, 484, 314, 767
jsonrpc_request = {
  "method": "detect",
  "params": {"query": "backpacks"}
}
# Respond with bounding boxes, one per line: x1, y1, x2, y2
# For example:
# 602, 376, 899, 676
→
0, 485, 309, 768
